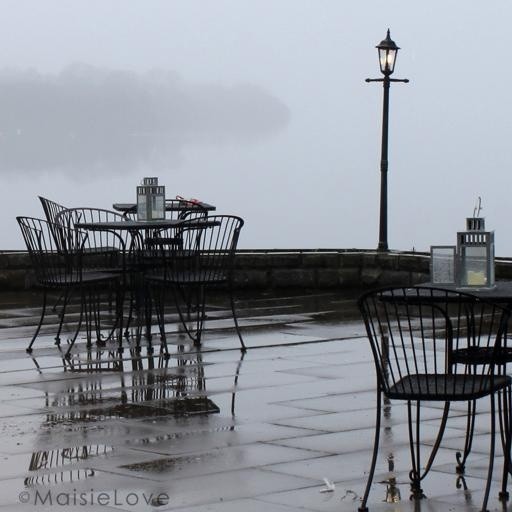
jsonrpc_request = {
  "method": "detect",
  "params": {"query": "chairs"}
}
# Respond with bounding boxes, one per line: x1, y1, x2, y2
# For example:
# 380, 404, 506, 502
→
15, 195, 247, 361
356, 275, 512, 512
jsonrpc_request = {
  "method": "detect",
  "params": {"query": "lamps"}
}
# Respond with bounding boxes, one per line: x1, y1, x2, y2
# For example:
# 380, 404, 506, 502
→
455, 217, 497, 290
136, 177, 166, 222
